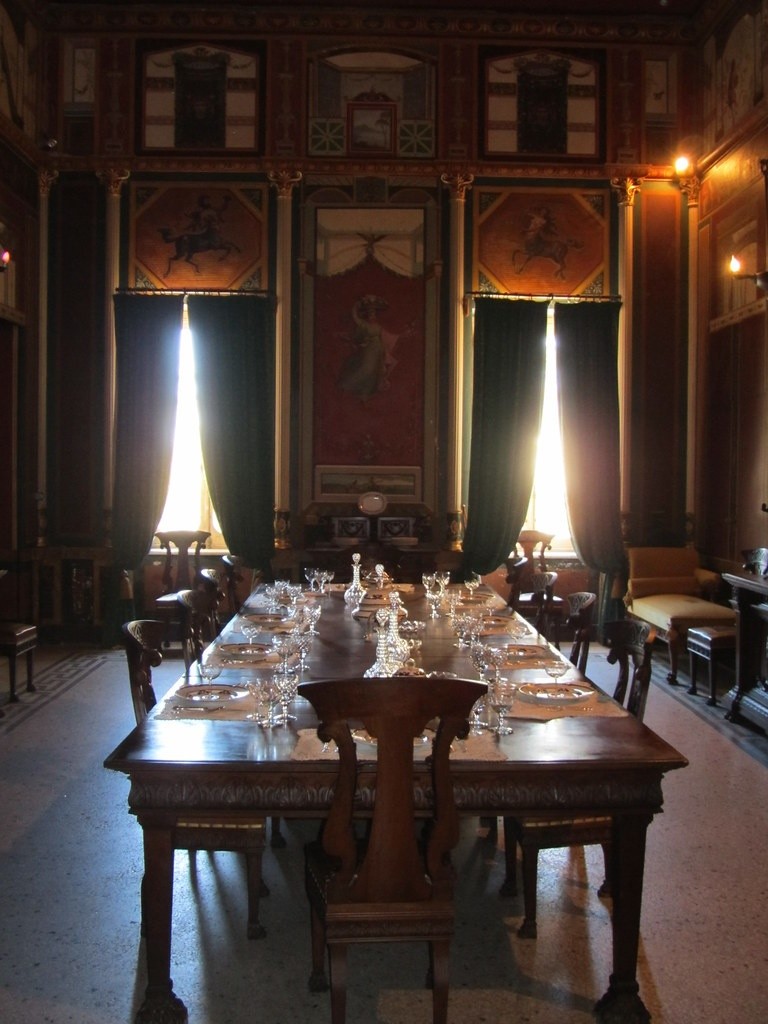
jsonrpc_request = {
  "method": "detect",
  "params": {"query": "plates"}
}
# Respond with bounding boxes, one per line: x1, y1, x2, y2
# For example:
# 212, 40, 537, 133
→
473, 615, 514, 626
353, 730, 428, 746
242, 613, 289, 627
219, 643, 274, 660
491, 643, 547, 659
462, 591, 498, 603
323, 580, 348, 593
518, 684, 595, 701
174, 684, 251, 704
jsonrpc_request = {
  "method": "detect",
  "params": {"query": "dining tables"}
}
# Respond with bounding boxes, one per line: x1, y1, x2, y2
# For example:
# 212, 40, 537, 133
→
102, 584, 689, 1024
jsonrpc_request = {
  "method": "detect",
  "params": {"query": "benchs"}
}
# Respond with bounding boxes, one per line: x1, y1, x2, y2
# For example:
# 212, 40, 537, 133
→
625, 591, 736, 682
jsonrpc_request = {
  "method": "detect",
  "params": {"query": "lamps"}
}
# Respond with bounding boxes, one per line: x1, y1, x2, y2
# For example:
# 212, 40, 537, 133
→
725, 255, 768, 290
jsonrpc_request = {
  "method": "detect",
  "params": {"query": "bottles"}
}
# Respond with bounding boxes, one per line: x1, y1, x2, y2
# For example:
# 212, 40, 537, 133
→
364, 591, 409, 678
345, 553, 367, 605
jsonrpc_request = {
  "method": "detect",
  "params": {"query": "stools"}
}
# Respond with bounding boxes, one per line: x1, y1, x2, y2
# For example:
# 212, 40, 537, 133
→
0, 622, 36, 702
686, 624, 736, 704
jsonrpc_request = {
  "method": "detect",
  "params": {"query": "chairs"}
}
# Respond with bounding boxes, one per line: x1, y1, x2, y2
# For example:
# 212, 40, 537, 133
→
120, 534, 718, 1024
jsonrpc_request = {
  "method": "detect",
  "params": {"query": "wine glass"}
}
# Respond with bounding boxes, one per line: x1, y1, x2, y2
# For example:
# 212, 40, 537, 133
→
196, 663, 224, 699
469, 648, 490, 681
544, 661, 569, 697
488, 648, 509, 683
491, 681, 517, 736
423, 571, 495, 648
505, 620, 528, 653
241, 568, 335, 728
467, 696, 487, 736
